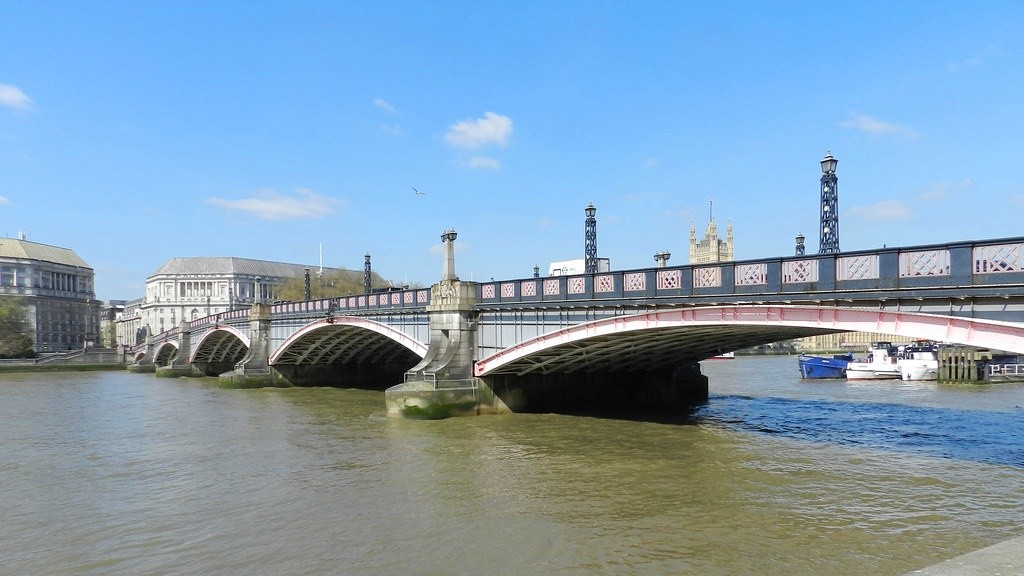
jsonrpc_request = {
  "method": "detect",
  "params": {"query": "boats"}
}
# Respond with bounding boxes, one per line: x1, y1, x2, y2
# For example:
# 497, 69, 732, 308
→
713, 351, 735, 359
846, 348, 902, 381
899, 350, 939, 382
797, 351, 855, 380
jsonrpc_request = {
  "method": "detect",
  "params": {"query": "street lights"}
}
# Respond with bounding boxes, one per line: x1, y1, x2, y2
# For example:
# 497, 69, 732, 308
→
304, 268, 310, 300
584, 201, 599, 274
795, 231, 806, 281
818, 149, 841, 254
363, 251, 372, 293
